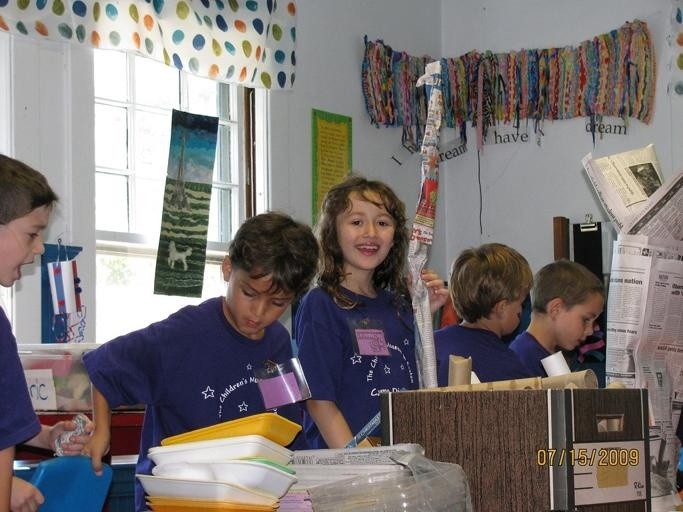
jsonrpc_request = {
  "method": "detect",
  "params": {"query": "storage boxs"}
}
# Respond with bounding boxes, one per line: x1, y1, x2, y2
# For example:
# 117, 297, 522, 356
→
312, 458, 469, 512
16, 339, 102, 413
378, 379, 651, 512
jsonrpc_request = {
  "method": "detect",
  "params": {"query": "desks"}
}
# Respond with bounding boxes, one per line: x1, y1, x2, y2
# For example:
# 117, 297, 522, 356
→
8, 410, 146, 460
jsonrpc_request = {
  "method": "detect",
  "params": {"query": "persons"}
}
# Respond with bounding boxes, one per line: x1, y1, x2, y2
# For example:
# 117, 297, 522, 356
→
0, 153, 97, 512
81, 210, 319, 512
429, 242, 533, 389
294, 177, 451, 450
506, 258, 605, 380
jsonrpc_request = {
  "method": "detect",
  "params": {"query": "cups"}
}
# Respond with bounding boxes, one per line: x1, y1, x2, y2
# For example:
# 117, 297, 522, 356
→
540, 350, 571, 378
572, 369, 601, 390
